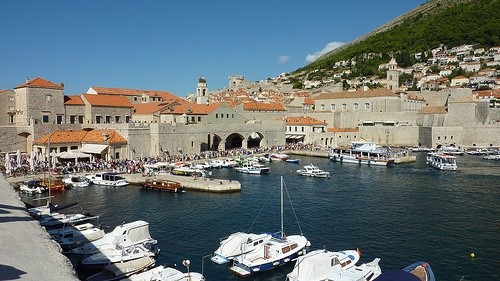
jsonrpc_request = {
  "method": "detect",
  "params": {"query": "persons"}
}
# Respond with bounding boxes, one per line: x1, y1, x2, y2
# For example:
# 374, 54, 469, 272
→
1, 138, 413, 182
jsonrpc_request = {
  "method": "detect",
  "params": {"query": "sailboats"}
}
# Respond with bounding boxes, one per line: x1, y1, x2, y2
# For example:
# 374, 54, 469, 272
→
228, 175, 312, 278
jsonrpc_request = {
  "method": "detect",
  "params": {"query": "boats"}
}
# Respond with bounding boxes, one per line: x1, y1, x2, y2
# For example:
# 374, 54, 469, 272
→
296, 164, 331, 178
373, 261, 437, 281
36, 177, 66, 190
286, 158, 299, 163
88, 173, 130, 187
140, 179, 185, 193
416, 143, 500, 161
426, 153, 458, 171
210, 230, 271, 265
171, 152, 289, 177
326, 257, 382, 281
64, 175, 89, 188
19, 179, 48, 193
27, 203, 205, 281
285, 247, 361, 281
